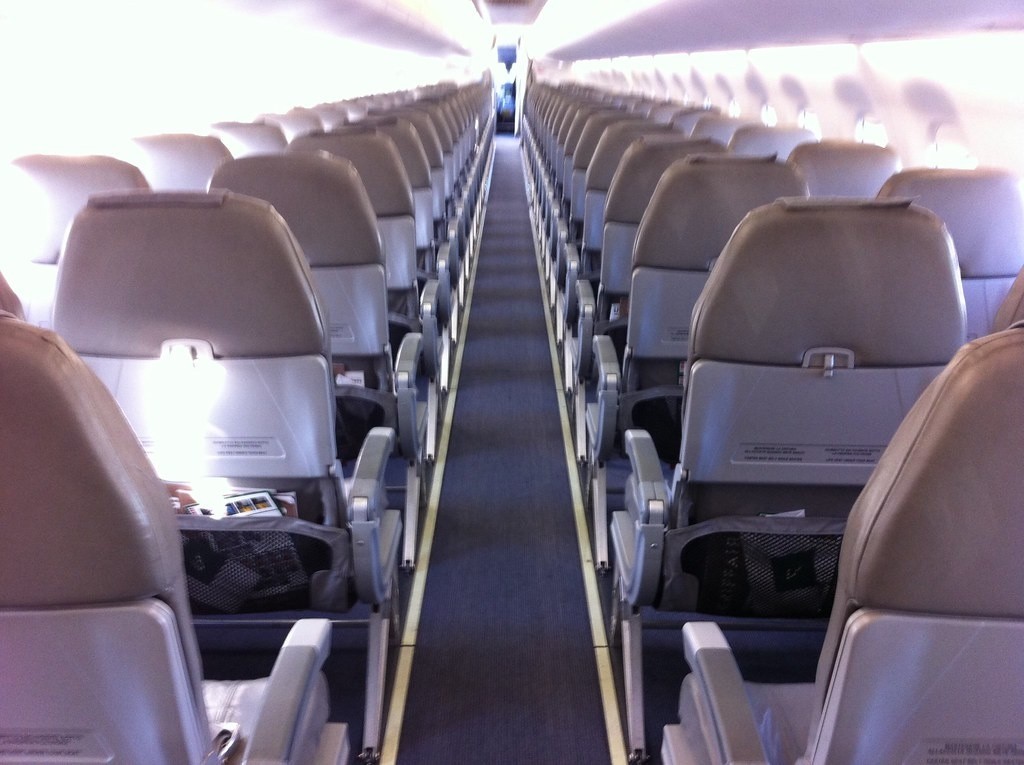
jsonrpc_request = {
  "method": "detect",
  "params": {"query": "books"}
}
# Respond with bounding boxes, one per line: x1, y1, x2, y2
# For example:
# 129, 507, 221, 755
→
329, 362, 370, 454
171, 487, 314, 616
609, 295, 629, 326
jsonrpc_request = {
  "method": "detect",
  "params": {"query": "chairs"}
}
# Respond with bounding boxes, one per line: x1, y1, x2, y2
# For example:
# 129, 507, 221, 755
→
519, 80, 1024, 765
0, 81, 492, 765
